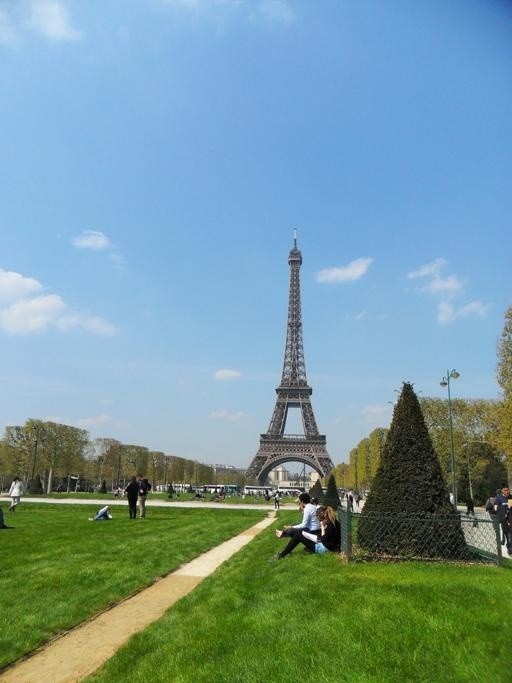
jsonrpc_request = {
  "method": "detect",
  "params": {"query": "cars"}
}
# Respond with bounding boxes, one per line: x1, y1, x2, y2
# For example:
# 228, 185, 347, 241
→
338, 488, 369, 501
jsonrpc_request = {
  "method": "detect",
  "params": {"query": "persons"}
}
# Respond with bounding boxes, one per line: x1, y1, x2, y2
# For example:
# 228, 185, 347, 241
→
8, 477, 23, 512
89, 506, 112, 521
486, 484, 512, 555
450, 493, 455, 504
341, 489, 360, 512
125, 473, 151, 519
466, 494, 474, 514
275, 494, 279, 509
276, 494, 341, 559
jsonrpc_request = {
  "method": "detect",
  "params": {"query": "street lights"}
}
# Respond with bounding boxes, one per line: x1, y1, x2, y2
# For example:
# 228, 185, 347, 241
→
440, 368, 461, 512
116, 445, 126, 488
30, 425, 45, 481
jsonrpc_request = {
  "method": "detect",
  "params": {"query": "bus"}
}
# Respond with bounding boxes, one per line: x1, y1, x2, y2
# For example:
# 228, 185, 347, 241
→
156, 482, 327, 497
